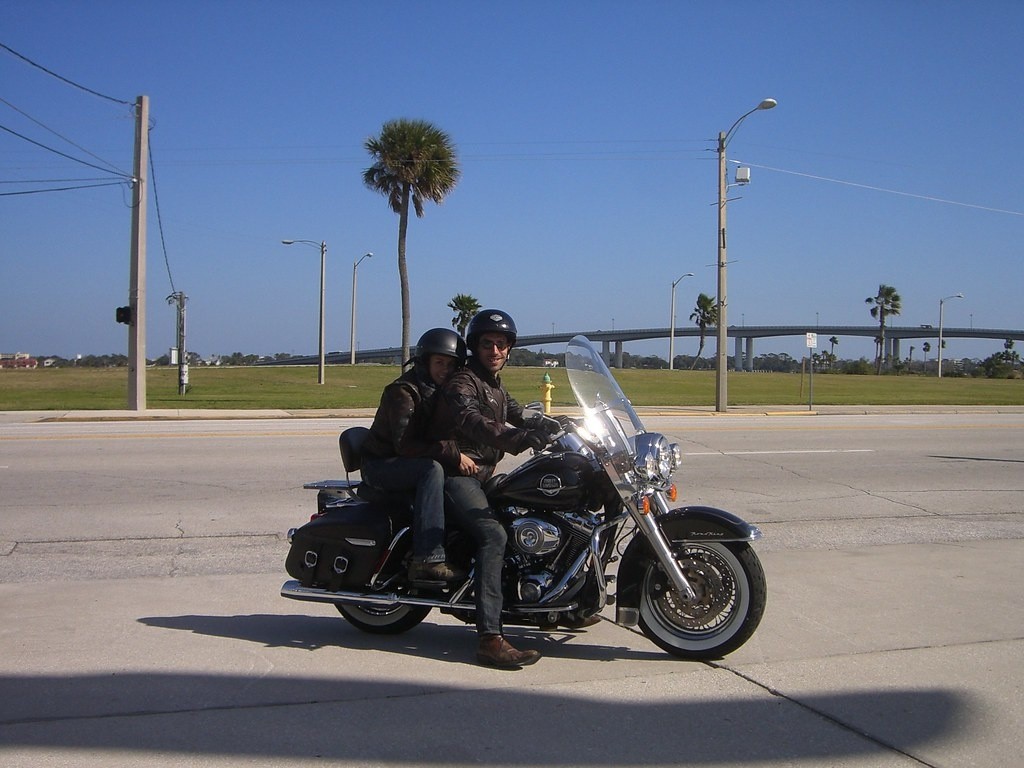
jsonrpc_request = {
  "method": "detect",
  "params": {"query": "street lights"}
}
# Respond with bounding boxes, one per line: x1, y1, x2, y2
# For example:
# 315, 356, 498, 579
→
552, 323, 554, 335
669, 272, 695, 370
282, 239, 326, 385
612, 318, 615, 331
937, 294, 964, 377
741, 313, 744, 327
715, 95, 778, 411
349, 252, 375, 364
816, 312, 819, 328
969, 313, 973, 330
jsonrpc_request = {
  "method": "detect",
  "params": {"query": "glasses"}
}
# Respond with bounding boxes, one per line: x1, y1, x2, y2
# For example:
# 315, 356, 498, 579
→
477, 339, 512, 351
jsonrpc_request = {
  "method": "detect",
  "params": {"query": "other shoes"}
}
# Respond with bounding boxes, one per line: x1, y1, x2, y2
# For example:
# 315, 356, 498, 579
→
407, 561, 465, 580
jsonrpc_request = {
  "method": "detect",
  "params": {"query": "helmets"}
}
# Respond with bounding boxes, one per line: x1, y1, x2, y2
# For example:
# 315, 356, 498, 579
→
414, 328, 467, 375
465, 309, 516, 354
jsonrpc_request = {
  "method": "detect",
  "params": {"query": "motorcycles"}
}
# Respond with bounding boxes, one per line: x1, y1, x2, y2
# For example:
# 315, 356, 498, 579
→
283, 337, 767, 662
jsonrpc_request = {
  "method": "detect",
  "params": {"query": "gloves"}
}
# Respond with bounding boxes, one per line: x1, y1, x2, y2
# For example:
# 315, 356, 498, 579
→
519, 428, 552, 451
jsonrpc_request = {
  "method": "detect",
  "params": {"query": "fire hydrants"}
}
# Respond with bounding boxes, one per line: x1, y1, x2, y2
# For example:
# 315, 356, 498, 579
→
538, 371, 555, 415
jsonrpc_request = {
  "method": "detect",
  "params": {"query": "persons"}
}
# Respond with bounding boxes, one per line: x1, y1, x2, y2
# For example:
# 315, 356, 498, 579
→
363, 329, 477, 581
432, 311, 579, 671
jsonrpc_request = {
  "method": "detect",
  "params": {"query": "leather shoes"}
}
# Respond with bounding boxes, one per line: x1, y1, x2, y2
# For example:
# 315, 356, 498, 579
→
476, 636, 542, 666
540, 616, 602, 632
